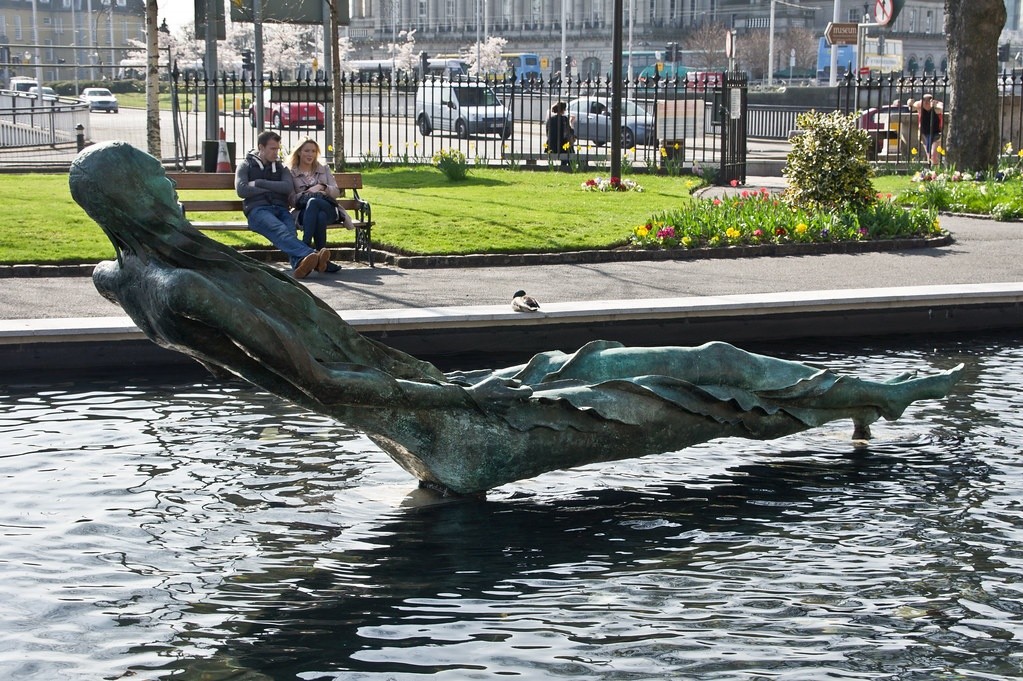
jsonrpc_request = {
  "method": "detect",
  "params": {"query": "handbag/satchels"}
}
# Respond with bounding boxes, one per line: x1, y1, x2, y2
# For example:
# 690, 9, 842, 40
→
295, 191, 338, 210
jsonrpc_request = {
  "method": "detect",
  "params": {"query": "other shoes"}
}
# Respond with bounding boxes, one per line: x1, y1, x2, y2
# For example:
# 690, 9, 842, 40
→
316, 247, 330, 273
315, 261, 342, 272
293, 253, 319, 278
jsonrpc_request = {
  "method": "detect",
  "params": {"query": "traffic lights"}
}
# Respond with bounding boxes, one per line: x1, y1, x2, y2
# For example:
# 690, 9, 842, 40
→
665, 45, 673, 62
676, 45, 682, 61
241, 49, 251, 69
423, 52, 431, 75
998, 42, 1010, 61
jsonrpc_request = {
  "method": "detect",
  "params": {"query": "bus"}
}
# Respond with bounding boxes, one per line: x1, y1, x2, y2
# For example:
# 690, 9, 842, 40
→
815, 36, 904, 82
608, 51, 740, 85
435, 52, 542, 86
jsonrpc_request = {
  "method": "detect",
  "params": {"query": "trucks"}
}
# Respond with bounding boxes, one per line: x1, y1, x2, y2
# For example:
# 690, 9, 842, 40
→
686, 71, 722, 90
9, 76, 38, 92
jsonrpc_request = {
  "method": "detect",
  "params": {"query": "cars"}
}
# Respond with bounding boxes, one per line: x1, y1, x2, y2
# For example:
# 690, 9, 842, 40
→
79, 87, 118, 113
546, 97, 655, 148
859, 99, 918, 152
248, 89, 325, 130
28, 87, 59, 101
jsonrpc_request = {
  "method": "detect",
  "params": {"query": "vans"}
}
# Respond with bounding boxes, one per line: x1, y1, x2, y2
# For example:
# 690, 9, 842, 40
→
413, 76, 513, 139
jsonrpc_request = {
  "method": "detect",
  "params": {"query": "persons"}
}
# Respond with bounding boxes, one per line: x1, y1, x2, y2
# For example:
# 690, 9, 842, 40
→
544, 103, 576, 166
235, 131, 330, 278
601, 105, 606, 115
287, 136, 342, 272
907, 95, 943, 168
69, 142, 964, 494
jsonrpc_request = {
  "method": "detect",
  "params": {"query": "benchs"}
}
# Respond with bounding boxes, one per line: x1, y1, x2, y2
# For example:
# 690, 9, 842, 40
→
167, 171, 377, 271
504, 152, 611, 166
